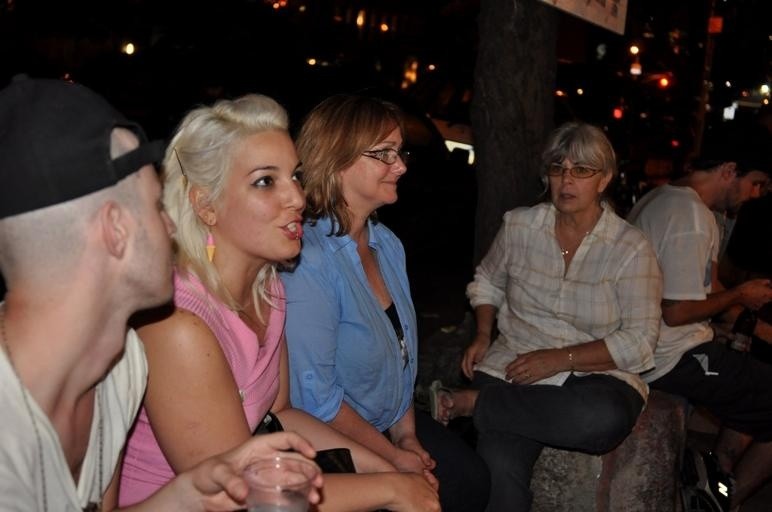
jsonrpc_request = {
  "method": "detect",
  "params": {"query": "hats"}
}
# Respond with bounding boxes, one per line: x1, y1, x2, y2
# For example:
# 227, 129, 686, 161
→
0, 74, 167, 218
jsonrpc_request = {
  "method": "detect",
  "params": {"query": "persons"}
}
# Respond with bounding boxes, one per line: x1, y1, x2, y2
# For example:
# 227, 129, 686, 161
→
0, 80, 324, 512
277, 88, 493, 512
626, 132, 772, 512
428, 122, 664, 512
116, 88, 443, 512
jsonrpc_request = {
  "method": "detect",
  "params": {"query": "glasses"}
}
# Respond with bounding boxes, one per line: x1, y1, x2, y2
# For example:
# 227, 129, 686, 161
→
358, 147, 410, 165
543, 164, 604, 179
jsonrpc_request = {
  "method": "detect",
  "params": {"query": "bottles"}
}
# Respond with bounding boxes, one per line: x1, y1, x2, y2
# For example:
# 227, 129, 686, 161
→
732, 309, 759, 354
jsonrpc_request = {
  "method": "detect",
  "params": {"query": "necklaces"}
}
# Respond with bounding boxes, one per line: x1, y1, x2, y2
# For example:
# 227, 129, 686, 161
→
560, 247, 570, 256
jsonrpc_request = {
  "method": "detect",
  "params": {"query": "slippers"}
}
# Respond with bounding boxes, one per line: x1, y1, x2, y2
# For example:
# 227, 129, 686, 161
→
429, 379, 454, 423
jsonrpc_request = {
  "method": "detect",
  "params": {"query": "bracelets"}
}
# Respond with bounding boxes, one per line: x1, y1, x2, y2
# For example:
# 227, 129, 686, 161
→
566, 347, 574, 371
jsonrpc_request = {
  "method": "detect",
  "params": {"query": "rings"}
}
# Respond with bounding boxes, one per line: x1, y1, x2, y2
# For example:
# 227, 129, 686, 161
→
524, 370, 532, 378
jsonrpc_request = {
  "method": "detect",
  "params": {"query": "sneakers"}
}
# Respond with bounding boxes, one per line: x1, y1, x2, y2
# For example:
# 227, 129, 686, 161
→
680, 446, 737, 512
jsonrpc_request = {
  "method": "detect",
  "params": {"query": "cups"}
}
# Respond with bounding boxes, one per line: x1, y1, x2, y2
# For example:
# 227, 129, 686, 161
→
241, 455, 314, 511
